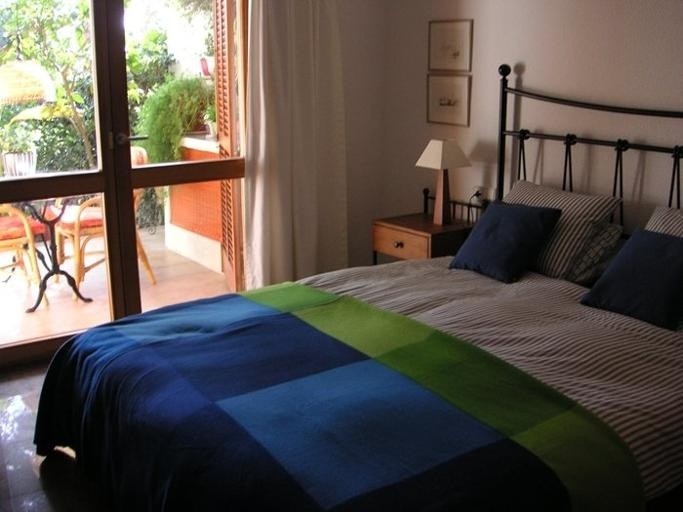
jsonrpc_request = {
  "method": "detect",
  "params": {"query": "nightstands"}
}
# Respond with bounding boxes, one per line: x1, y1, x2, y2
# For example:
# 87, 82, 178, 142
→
370, 214, 473, 265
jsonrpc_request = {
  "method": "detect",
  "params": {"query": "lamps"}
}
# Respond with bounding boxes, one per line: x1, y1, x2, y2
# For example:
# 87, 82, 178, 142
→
414, 137, 471, 225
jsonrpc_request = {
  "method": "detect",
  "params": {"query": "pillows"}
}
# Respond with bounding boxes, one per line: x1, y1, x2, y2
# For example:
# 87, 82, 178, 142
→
448, 180, 683, 331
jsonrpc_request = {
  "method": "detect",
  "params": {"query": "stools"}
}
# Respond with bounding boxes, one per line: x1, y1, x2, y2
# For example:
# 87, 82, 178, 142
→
0, 204, 50, 306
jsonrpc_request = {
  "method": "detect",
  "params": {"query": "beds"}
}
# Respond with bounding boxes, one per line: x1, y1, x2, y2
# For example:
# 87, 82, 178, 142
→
33, 64, 683, 511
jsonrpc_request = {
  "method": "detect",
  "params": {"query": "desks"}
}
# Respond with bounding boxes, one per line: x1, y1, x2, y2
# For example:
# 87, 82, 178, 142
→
21, 195, 93, 313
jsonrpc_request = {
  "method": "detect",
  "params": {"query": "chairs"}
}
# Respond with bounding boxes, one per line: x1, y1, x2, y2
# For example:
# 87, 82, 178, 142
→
42, 147, 156, 300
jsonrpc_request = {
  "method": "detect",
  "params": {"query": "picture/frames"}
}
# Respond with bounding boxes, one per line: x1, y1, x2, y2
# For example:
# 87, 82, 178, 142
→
426, 74, 471, 127
428, 19, 473, 72
